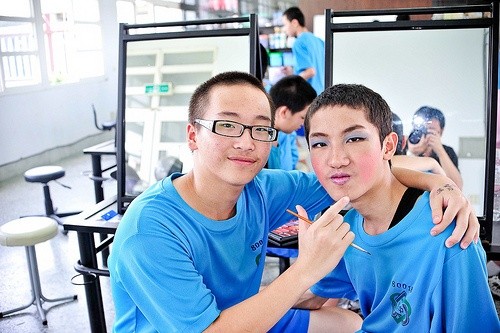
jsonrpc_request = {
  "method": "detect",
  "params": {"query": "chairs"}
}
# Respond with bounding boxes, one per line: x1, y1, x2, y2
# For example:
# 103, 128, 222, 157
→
92, 102, 117, 133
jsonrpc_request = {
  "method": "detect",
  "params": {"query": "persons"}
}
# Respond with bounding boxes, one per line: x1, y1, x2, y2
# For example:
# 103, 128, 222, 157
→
263, 74, 317, 170
278, 5, 324, 136
103, 68, 482, 333
391, 113, 407, 155
258, 84, 500, 333
389, 155, 447, 176
403, 106, 464, 191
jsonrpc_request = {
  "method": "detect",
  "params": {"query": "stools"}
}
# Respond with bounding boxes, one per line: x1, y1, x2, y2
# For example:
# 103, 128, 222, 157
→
20, 166, 85, 236
1, 216, 78, 326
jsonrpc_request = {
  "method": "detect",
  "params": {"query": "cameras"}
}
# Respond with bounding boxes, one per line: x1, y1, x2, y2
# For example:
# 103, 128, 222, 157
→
409, 127, 427, 144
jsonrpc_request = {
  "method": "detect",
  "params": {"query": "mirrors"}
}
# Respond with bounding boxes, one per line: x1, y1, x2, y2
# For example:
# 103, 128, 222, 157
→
117, 14, 260, 214
324, 4, 499, 247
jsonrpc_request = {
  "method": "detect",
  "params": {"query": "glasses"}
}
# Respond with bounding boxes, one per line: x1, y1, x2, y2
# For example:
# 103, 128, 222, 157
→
194, 117, 279, 142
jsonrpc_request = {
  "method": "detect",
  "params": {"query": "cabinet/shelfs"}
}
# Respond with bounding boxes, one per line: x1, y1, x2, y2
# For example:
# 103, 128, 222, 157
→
259, 25, 298, 83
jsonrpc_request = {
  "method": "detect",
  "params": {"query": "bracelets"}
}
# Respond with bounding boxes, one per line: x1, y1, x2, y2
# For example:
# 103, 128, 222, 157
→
406, 150, 415, 156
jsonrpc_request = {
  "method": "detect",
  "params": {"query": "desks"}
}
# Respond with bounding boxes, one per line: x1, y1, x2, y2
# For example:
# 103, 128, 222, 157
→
61, 192, 500, 333
83, 139, 117, 204
